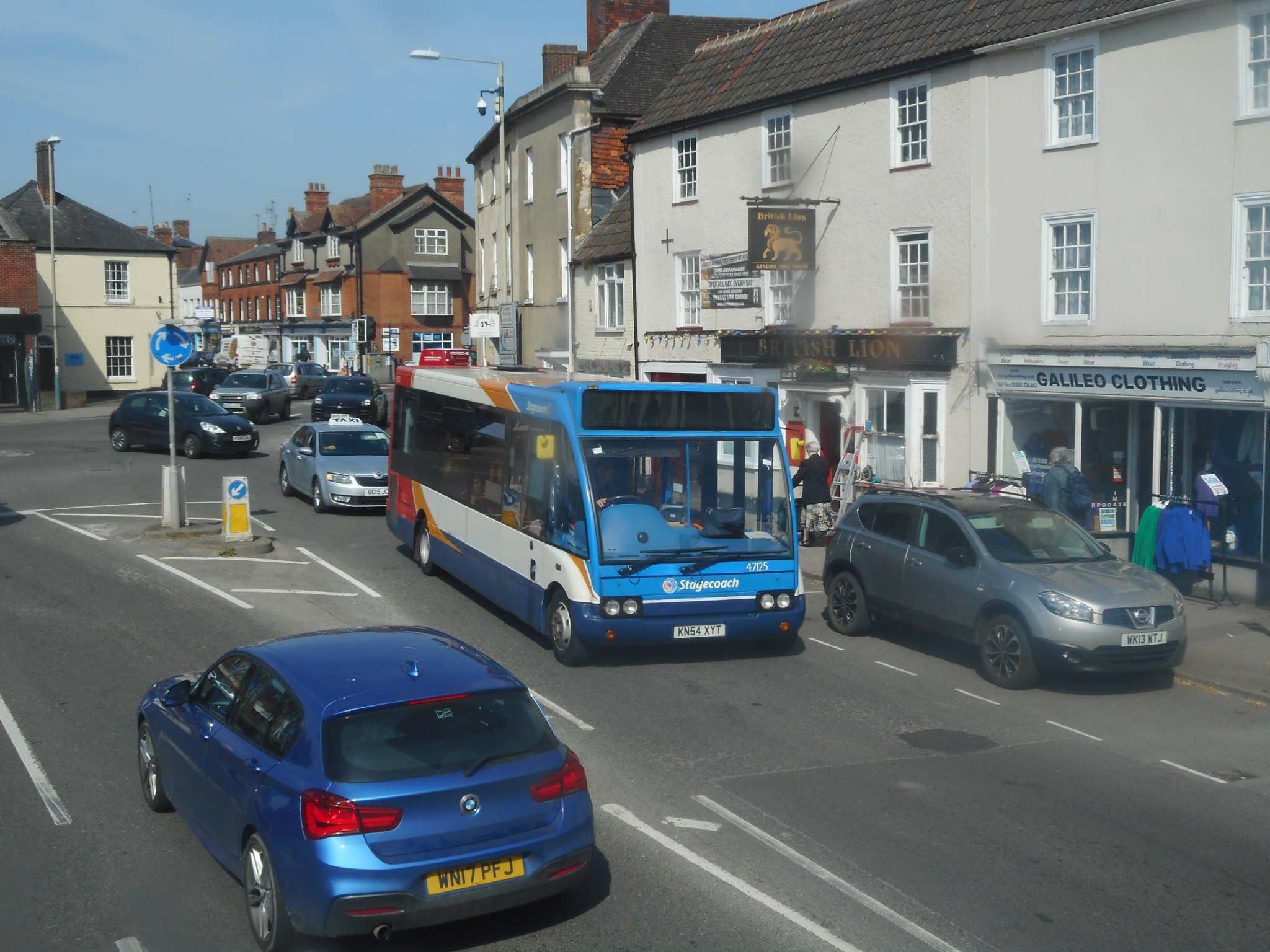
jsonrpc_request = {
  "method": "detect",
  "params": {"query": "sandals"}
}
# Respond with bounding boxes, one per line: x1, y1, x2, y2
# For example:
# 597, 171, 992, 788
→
800, 540, 810, 547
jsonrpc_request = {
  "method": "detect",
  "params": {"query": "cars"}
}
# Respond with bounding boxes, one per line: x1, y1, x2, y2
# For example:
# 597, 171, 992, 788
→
277, 417, 392, 514
135, 624, 597, 952
159, 349, 233, 398
820, 487, 1190, 692
106, 389, 260, 459
309, 375, 388, 427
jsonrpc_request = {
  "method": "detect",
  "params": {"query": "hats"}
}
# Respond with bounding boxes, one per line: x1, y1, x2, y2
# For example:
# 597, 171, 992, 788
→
1227, 525, 1242, 538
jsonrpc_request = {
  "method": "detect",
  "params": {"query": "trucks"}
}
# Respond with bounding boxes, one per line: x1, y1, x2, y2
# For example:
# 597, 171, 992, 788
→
212, 333, 270, 371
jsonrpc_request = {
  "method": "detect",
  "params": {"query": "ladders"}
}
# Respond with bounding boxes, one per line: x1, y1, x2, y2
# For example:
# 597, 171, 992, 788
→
826, 426, 865, 528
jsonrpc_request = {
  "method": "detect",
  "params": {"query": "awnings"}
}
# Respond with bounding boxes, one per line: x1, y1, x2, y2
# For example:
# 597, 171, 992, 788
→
312, 269, 343, 283
278, 273, 307, 286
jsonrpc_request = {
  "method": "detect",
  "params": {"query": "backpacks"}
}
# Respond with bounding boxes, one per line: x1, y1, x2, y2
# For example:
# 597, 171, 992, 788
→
1056, 464, 1093, 513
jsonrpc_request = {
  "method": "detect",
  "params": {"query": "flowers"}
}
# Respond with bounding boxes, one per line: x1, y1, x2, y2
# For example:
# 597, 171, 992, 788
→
791, 357, 837, 374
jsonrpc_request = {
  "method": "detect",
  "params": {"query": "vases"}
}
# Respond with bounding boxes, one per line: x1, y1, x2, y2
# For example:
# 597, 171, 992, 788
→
795, 373, 837, 383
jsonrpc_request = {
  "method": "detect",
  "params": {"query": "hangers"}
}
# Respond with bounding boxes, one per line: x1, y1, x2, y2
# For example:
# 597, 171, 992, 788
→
974, 471, 1022, 488
1159, 495, 1192, 508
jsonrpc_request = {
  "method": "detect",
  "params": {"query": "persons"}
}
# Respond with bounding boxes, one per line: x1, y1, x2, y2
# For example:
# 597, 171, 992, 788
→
590, 461, 638, 510
158, 396, 185, 421
1042, 447, 1093, 549
322, 434, 328, 444
232, 377, 247, 386
256, 375, 266, 388
321, 434, 338, 454
338, 363, 352, 376
298, 344, 312, 362
470, 474, 485, 507
791, 441, 834, 547
269, 340, 280, 369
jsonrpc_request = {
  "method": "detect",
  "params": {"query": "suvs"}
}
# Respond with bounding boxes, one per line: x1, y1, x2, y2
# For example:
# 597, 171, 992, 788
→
209, 368, 291, 426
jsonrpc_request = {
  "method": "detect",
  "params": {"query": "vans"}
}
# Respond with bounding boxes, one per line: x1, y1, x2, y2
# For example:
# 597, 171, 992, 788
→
267, 361, 336, 401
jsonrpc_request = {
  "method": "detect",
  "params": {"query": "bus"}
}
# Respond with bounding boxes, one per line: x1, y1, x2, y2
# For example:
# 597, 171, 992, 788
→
388, 367, 807, 668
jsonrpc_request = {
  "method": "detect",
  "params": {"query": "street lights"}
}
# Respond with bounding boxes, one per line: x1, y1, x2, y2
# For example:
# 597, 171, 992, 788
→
46, 133, 62, 413
410, 47, 510, 306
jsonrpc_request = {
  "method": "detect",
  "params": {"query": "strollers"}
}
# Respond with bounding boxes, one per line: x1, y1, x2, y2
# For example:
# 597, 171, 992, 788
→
777, 484, 803, 533
